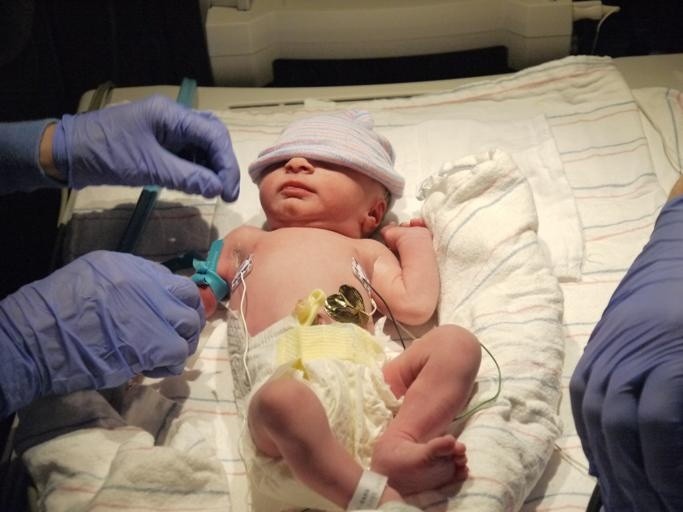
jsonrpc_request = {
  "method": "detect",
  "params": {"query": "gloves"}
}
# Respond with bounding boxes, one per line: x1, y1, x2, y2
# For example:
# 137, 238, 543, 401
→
0, 250, 206, 418
51, 96, 240, 202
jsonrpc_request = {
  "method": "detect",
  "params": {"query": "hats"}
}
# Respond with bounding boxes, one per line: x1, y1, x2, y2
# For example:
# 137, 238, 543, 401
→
248, 109, 405, 197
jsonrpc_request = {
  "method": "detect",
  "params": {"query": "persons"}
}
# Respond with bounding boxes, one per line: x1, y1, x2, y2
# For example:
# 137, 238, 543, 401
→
189, 113, 481, 512
0, 95, 240, 420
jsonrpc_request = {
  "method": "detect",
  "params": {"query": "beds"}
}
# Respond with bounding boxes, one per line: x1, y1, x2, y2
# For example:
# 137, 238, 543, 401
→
0, 53, 683, 512
